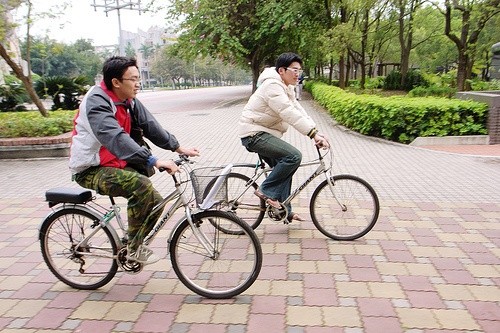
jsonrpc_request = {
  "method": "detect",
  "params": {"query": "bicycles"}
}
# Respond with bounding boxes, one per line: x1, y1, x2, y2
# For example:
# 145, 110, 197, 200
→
38, 151, 263, 299
202, 136, 380, 241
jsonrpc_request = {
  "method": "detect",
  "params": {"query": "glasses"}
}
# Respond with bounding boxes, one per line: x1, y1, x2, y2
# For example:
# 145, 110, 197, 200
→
122, 76, 142, 83
286, 67, 304, 75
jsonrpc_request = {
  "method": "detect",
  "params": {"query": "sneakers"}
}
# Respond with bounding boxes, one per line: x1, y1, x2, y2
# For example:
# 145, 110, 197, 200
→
296, 97, 302, 101
126, 247, 161, 264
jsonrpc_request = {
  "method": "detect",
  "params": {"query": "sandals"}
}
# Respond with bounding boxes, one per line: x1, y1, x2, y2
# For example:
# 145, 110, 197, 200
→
254, 189, 281, 210
289, 211, 306, 222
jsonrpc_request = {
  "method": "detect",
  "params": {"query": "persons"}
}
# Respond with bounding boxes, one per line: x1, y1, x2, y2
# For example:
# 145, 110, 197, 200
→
296, 73, 303, 100
239, 53, 331, 222
68, 56, 201, 265
